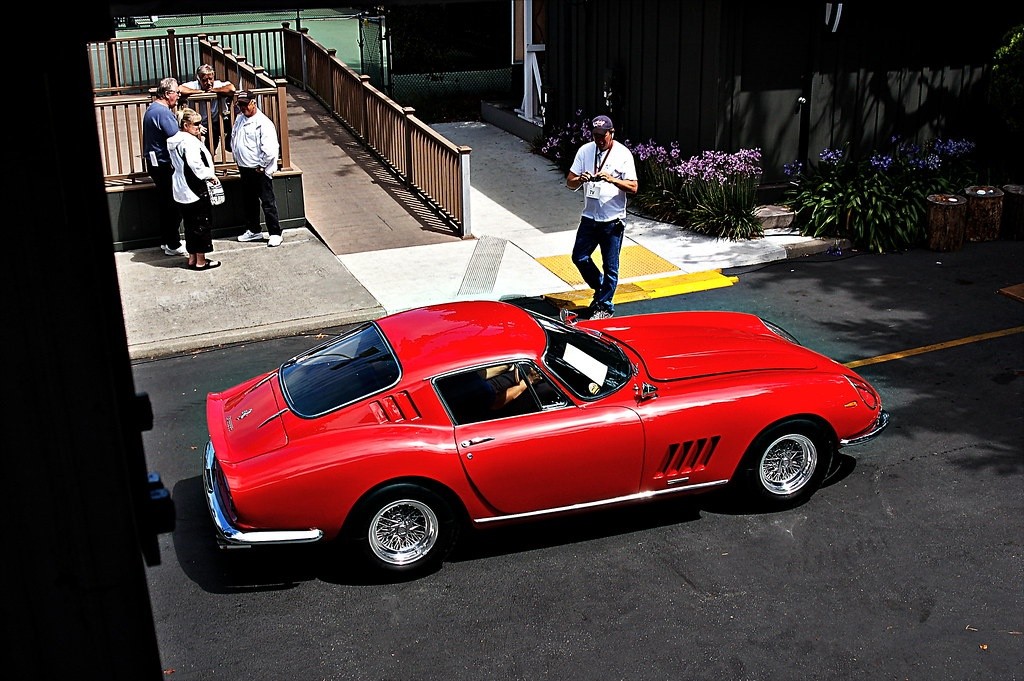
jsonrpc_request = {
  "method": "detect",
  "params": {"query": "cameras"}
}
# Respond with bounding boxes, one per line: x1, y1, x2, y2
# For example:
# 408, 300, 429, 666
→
589, 176, 600, 182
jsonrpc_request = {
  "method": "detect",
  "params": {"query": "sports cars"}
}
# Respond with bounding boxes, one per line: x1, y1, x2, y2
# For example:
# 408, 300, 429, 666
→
201, 300, 893, 577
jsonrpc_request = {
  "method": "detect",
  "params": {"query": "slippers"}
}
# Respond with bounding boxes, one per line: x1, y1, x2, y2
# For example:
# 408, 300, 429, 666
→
187, 258, 221, 270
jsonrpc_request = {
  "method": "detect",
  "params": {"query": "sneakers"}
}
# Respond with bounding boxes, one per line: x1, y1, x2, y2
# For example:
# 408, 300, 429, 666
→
238, 230, 263, 242
160, 240, 186, 250
164, 243, 189, 256
267, 235, 284, 247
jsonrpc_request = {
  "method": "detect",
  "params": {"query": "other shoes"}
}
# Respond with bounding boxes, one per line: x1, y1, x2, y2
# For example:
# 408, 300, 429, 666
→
588, 308, 615, 320
592, 276, 604, 299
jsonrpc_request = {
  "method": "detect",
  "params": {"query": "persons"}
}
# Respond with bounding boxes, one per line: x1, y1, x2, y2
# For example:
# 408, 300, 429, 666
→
440, 364, 539, 424
567, 116, 637, 322
143, 64, 284, 271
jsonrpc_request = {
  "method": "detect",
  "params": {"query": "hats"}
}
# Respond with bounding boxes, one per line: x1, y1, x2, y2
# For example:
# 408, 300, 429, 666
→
591, 115, 613, 135
235, 89, 256, 102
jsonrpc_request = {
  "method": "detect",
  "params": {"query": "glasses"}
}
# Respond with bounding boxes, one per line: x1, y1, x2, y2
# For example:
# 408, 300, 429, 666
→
236, 101, 249, 107
165, 90, 181, 94
190, 121, 202, 126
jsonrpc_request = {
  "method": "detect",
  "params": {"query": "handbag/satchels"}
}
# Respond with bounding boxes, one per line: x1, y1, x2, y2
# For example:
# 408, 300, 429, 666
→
205, 178, 225, 205
